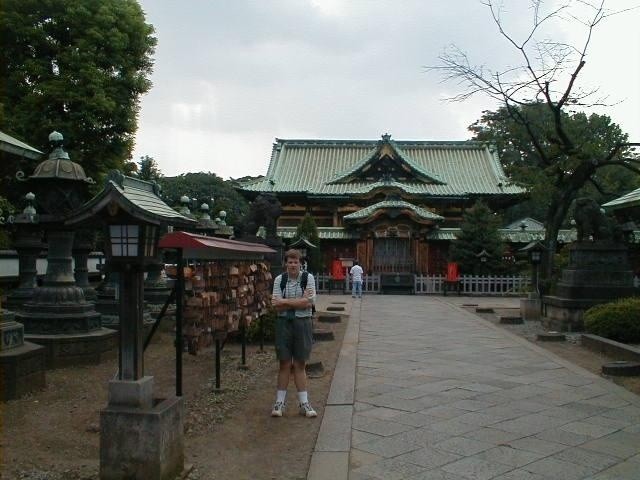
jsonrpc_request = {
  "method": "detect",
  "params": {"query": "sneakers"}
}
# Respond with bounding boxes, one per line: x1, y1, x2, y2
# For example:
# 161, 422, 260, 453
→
271, 401, 285, 416
299, 402, 317, 417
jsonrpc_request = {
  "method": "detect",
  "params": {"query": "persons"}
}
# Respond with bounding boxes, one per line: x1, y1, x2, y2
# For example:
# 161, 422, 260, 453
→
271, 247, 318, 417
349, 259, 365, 298
